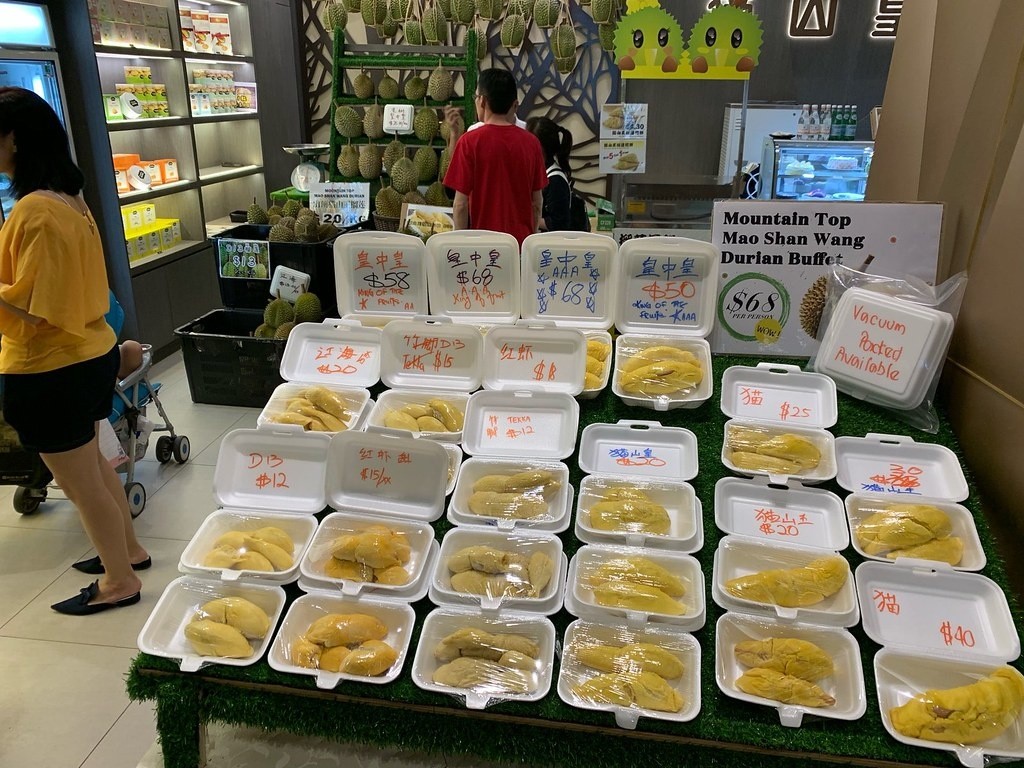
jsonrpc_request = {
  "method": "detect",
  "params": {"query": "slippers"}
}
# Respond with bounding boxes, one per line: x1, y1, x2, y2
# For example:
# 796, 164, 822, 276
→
51, 579, 140, 615
72, 555, 151, 574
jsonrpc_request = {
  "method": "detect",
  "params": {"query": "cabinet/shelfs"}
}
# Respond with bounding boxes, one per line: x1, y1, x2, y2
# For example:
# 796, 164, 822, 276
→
329, 26, 477, 199
758, 136, 875, 202
86, 0, 269, 365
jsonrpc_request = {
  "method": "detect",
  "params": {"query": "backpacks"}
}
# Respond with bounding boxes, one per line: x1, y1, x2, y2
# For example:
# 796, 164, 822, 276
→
546, 167, 591, 232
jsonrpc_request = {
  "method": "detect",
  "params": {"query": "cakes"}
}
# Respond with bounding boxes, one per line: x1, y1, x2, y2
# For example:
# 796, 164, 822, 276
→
785, 161, 814, 175
828, 157, 858, 169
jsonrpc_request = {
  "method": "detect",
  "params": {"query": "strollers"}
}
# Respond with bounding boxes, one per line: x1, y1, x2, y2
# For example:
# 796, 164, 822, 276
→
11, 290, 190, 517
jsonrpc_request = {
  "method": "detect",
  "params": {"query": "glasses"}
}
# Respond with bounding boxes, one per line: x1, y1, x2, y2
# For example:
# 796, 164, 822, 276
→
472, 94, 481, 101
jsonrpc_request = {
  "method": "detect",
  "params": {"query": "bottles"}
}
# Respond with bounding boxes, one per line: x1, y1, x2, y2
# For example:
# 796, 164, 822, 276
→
830, 105, 843, 141
841, 105, 851, 141
844, 105, 857, 141
819, 105, 831, 141
828, 105, 837, 141
819, 105, 826, 141
808, 105, 819, 141
797, 105, 809, 140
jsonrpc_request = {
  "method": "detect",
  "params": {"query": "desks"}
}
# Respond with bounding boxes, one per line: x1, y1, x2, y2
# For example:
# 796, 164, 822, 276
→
128, 342, 1024, 768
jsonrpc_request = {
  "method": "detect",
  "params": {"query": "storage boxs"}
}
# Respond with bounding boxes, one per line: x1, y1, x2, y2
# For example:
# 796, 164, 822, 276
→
208, 225, 346, 313
121, 201, 183, 262
174, 308, 322, 408
112, 151, 181, 191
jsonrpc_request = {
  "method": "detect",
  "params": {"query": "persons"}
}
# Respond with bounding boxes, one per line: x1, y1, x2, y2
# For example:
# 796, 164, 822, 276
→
442, 67, 550, 318
525, 115, 573, 234
0, 86, 154, 615
114, 340, 144, 394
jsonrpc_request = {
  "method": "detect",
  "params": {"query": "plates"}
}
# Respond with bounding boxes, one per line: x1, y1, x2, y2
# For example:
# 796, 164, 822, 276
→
769, 134, 796, 139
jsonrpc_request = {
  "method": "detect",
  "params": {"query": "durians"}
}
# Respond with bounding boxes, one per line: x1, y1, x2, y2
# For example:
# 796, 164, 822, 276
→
799, 256, 873, 340
254, 284, 322, 340
321, 0, 620, 217
399, 219, 438, 245
220, 242, 268, 278
183, 341, 1024, 745
248, 191, 337, 243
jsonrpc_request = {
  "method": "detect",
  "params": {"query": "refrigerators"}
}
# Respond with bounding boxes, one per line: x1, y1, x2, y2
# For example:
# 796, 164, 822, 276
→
0, 0, 83, 233
